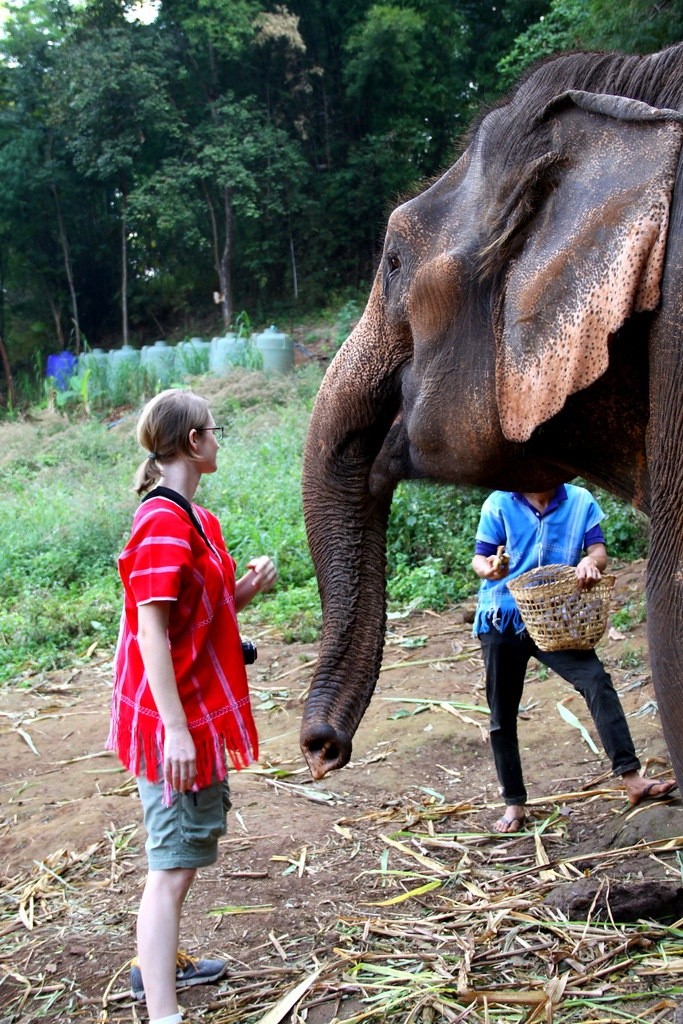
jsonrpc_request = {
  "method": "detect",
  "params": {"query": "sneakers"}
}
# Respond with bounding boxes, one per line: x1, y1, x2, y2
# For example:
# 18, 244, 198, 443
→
130, 948, 227, 1000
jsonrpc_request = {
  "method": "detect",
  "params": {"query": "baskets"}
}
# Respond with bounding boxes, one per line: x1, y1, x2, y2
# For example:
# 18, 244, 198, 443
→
506, 562, 616, 654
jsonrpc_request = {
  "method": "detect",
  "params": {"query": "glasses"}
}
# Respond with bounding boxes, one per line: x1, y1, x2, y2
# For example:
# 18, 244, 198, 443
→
193, 426, 224, 442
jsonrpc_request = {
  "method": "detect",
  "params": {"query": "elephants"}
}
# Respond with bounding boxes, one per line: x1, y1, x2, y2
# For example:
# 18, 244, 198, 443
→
290, 43, 683, 781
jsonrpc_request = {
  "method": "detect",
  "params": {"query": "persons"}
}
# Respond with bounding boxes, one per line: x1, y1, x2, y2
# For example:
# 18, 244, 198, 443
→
470, 483, 680, 834
104, 387, 279, 1024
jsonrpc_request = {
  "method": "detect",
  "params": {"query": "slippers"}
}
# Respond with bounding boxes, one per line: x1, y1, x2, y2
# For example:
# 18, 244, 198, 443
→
635, 776, 678, 807
492, 814, 527, 834
